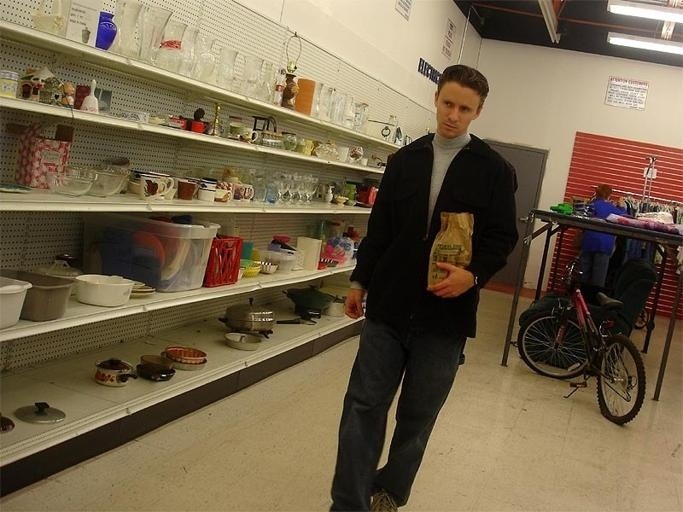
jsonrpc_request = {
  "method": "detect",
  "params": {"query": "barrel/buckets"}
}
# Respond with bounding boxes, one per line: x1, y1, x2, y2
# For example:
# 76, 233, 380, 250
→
297, 233, 323, 272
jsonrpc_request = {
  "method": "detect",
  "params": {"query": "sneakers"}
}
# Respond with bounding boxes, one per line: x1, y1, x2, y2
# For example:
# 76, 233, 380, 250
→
370, 488, 398, 512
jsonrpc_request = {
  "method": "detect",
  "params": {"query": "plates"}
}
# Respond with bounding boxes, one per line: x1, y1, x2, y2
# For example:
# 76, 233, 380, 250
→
130, 281, 156, 298
136, 364, 175, 382
136, 354, 174, 372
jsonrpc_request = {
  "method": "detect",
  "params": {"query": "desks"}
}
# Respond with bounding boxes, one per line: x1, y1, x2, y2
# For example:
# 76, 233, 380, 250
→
502, 209, 683, 401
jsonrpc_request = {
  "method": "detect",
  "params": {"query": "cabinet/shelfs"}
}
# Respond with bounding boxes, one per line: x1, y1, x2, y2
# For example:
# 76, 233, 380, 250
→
0, 18, 407, 498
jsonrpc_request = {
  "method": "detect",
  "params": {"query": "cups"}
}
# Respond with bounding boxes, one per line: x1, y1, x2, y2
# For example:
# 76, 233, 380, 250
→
140, 171, 174, 200
174, 176, 255, 202
242, 128, 258, 144
297, 138, 363, 163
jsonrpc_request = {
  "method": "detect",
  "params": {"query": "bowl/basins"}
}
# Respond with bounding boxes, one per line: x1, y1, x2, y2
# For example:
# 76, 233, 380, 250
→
238, 258, 279, 280
46, 165, 126, 198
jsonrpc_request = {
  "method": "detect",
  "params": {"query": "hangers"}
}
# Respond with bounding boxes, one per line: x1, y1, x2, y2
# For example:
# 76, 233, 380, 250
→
617, 192, 683, 212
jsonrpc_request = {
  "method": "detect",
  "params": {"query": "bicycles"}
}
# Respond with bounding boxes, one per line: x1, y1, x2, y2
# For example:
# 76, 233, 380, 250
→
519, 249, 647, 426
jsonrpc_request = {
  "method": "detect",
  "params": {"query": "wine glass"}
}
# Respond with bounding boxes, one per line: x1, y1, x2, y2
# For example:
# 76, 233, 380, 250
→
276, 174, 319, 205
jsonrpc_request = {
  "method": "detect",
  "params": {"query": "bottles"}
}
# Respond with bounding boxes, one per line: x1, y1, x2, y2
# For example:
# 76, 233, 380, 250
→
0, 70, 19, 99
306, 219, 340, 242
312, 81, 398, 144
260, 131, 296, 151
225, 116, 243, 139
273, 70, 286, 106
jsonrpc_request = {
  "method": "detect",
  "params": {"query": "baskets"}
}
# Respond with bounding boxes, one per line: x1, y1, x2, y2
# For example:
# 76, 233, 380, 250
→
204, 234, 243, 287
261, 117, 284, 149
166, 345, 208, 364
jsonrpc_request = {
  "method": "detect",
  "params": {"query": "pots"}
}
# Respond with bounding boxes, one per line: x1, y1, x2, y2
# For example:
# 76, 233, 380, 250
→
95, 360, 137, 387
282, 284, 332, 317
218, 297, 276, 333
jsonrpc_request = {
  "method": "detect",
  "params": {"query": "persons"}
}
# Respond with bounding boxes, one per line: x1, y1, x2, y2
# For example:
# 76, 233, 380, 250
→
329, 65, 519, 512
579, 184, 626, 285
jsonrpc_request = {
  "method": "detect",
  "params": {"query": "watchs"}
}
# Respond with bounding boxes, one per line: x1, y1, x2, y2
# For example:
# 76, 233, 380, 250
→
474, 275, 478, 285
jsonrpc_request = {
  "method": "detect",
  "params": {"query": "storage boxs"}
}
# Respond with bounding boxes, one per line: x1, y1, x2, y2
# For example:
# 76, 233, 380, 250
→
16, 121, 75, 190
0, 269, 77, 322
0, 276, 32, 330
83, 211, 220, 293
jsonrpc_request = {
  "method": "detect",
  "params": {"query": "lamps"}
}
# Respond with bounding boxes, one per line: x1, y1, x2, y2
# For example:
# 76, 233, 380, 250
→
607, 32, 683, 56
607, 0, 683, 23
